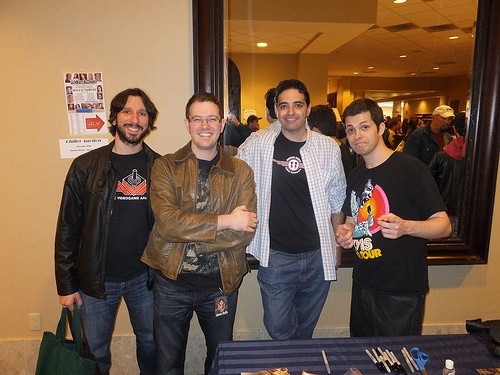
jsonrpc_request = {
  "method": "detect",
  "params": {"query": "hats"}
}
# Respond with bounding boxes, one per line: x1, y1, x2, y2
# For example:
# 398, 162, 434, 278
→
247, 115, 262, 121
433, 105, 456, 119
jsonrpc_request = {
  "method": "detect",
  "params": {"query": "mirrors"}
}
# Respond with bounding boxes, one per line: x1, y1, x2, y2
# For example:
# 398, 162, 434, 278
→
192, 0, 500, 270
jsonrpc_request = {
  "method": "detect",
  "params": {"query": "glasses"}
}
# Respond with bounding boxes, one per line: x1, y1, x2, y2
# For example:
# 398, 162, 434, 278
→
186, 115, 222, 123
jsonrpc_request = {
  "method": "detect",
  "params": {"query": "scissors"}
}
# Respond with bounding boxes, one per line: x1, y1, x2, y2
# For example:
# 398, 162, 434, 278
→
410, 347, 429, 375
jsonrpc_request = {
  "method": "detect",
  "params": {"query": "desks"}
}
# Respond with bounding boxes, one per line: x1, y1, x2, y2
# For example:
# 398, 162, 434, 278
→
209, 333, 500, 375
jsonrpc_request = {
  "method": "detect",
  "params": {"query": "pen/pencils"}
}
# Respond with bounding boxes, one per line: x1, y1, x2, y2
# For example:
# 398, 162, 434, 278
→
321, 350, 331, 375
365, 346, 421, 375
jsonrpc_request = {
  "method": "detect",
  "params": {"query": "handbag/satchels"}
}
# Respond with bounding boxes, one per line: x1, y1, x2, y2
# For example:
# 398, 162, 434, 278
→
35, 302, 99, 375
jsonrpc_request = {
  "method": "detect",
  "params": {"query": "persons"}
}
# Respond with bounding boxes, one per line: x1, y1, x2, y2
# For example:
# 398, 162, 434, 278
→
140, 92, 259, 375
64, 73, 105, 110
335, 97, 453, 338
224, 103, 469, 215
232, 79, 347, 340
54, 88, 165, 375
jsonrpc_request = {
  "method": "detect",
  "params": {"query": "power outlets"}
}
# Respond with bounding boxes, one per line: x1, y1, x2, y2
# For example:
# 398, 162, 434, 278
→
28, 313, 42, 331
